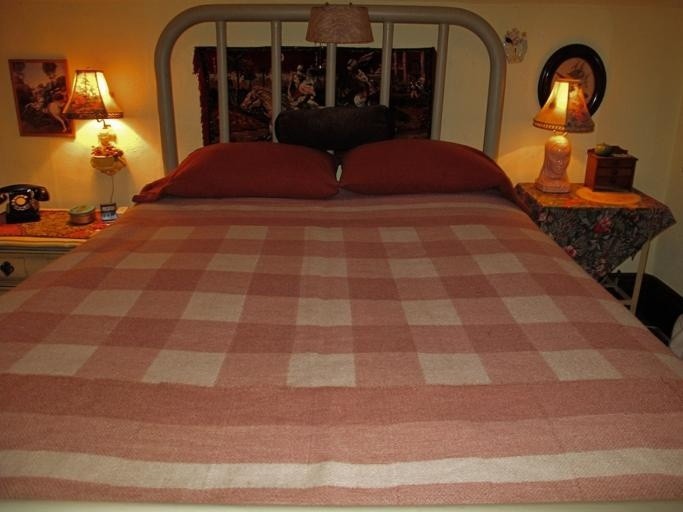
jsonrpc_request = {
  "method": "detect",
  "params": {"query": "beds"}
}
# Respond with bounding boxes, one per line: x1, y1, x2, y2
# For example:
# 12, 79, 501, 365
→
0, 1, 682, 511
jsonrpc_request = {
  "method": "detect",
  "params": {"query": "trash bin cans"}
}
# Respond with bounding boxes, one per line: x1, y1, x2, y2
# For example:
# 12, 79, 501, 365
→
597, 272, 683, 347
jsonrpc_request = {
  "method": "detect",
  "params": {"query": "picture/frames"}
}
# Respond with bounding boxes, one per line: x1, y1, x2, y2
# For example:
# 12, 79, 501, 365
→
537, 44, 606, 117
6, 58, 73, 139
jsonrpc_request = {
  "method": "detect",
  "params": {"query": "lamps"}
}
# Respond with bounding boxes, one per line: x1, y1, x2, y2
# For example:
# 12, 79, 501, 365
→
532, 79, 596, 193
302, 0, 376, 45
64, 68, 126, 177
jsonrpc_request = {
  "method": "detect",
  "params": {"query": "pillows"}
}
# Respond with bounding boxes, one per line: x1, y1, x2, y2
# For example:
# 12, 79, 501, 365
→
135, 144, 340, 201
341, 137, 514, 199
272, 106, 407, 144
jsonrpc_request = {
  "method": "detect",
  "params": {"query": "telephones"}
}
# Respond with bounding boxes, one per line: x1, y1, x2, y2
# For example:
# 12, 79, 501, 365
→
0, 184, 49, 224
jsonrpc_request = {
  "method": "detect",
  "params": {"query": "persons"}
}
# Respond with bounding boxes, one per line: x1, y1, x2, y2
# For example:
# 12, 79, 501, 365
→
8, 61, 72, 135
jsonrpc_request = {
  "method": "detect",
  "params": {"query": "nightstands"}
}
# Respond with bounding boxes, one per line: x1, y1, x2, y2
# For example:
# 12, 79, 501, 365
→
515, 176, 676, 316
0, 201, 126, 285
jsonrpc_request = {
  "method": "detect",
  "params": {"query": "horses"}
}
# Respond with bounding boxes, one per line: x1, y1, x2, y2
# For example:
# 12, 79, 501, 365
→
240, 85, 287, 116
